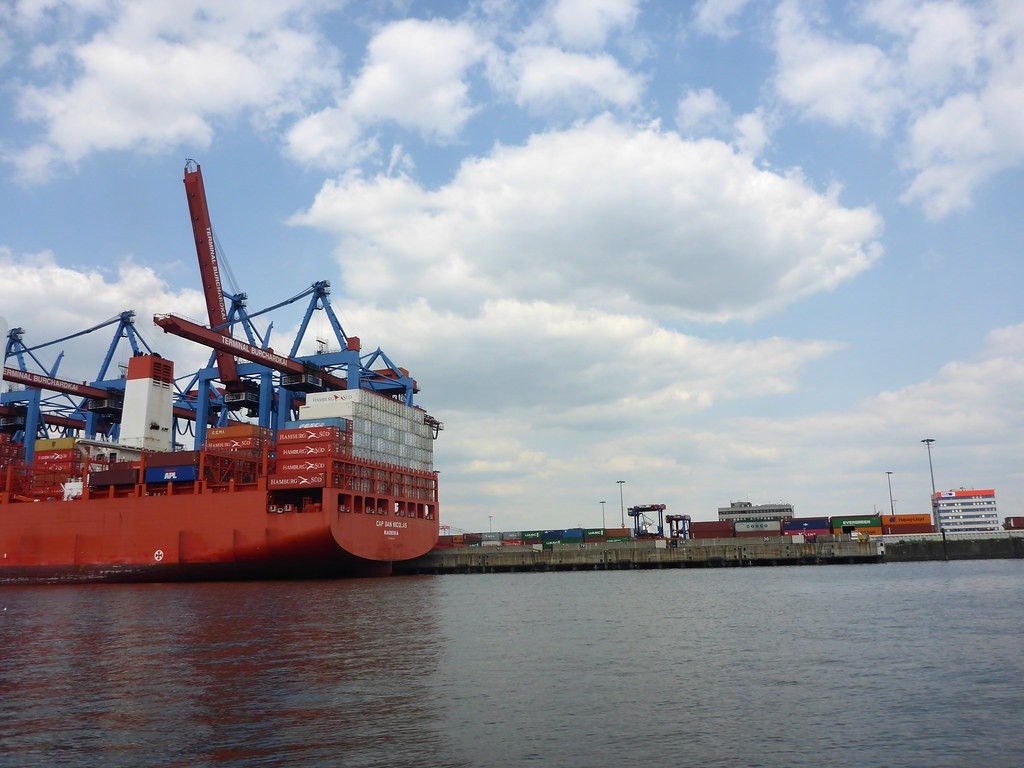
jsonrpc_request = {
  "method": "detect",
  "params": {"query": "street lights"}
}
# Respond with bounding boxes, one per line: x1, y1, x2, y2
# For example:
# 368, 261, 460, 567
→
921, 439, 941, 533
616, 481, 626, 528
487, 515, 494, 532
600, 500, 606, 529
884, 471, 897, 515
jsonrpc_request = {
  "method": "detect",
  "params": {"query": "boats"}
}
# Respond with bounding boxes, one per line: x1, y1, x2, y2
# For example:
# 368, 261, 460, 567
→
0, 448, 441, 585
